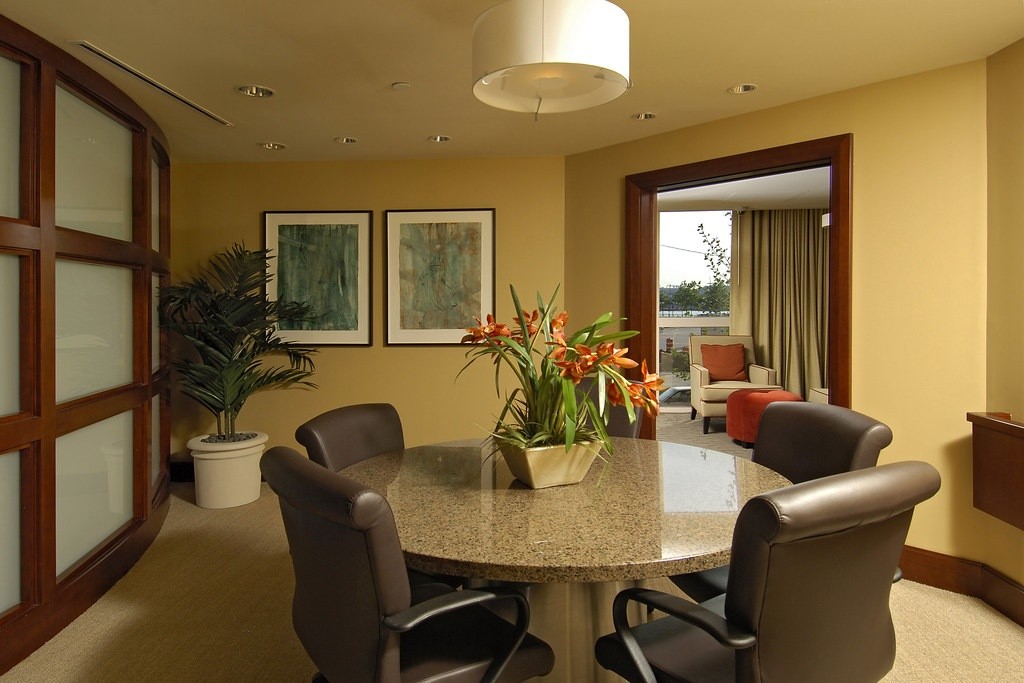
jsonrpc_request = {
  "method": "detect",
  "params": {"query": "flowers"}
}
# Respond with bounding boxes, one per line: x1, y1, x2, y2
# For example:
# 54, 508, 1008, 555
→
453, 283, 666, 469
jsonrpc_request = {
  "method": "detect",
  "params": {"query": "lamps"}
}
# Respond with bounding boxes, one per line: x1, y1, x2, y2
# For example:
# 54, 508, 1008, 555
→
470, 0, 633, 122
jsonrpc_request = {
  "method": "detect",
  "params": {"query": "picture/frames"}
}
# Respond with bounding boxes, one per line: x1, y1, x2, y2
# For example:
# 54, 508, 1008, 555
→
261, 210, 374, 348
383, 208, 496, 347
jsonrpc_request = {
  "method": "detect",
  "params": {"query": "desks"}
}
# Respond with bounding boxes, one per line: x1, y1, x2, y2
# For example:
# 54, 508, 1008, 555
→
334, 437, 794, 683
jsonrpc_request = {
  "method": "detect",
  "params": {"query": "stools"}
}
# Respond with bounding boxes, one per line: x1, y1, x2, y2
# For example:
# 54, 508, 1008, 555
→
725, 388, 803, 448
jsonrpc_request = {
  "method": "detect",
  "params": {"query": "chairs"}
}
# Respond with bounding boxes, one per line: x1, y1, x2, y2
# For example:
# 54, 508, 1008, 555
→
665, 400, 892, 606
594, 460, 941, 683
258, 445, 555, 683
688, 335, 784, 435
294, 404, 462, 590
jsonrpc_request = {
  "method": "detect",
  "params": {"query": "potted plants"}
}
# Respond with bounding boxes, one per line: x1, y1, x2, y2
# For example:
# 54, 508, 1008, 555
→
155, 239, 319, 510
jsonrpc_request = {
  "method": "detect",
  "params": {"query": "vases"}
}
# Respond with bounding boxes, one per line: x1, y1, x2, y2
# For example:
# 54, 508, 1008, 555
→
491, 431, 605, 490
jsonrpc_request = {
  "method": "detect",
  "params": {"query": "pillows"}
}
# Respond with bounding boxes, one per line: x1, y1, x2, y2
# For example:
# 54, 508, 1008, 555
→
701, 343, 750, 381
